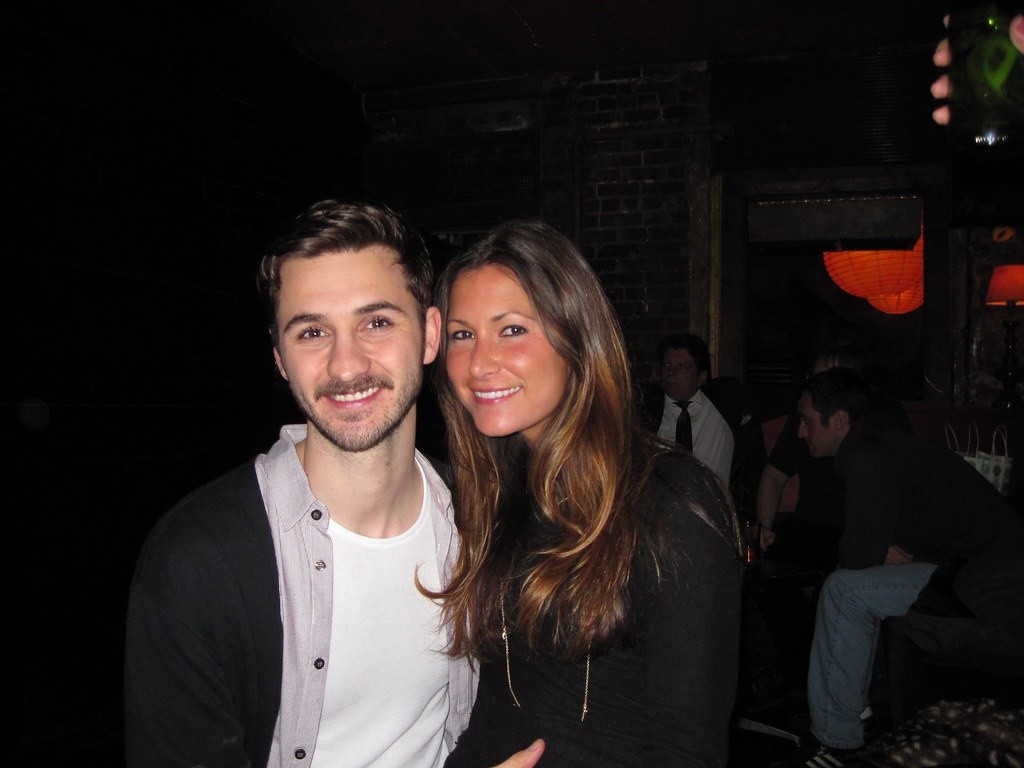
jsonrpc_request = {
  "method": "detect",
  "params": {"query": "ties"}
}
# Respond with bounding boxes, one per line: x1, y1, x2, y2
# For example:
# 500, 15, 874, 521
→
672, 401, 694, 455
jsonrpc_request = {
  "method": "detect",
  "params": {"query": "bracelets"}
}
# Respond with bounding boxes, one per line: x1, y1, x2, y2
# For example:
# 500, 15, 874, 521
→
742, 519, 753, 528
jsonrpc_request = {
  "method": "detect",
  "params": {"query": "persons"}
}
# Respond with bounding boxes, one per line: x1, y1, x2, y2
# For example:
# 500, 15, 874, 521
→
641, 332, 768, 538
409, 220, 743, 768
740, 337, 916, 701
123, 199, 479, 768
788, 364, 1024, 768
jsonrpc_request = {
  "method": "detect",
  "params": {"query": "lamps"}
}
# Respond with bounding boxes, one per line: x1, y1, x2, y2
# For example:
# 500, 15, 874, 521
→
982, 263, 1024, 410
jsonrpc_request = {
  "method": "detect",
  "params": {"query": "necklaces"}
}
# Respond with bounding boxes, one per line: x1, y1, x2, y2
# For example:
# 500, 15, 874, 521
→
499, 581, 592, 722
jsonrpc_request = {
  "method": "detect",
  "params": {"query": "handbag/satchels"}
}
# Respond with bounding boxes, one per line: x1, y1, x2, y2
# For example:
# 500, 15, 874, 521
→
942, 420, 1011, 497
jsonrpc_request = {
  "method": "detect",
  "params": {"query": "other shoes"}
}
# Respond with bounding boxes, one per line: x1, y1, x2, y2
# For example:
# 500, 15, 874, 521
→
804, 751, 845, 768
